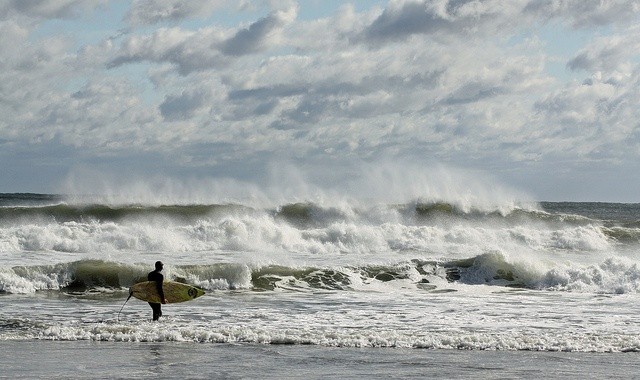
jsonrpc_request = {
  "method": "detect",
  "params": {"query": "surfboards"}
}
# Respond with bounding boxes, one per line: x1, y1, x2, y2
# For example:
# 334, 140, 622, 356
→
129, 281, 205, 304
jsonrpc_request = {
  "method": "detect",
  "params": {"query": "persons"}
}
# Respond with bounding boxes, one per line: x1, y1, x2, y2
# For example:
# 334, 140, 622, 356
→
148, 261, 168, 321
